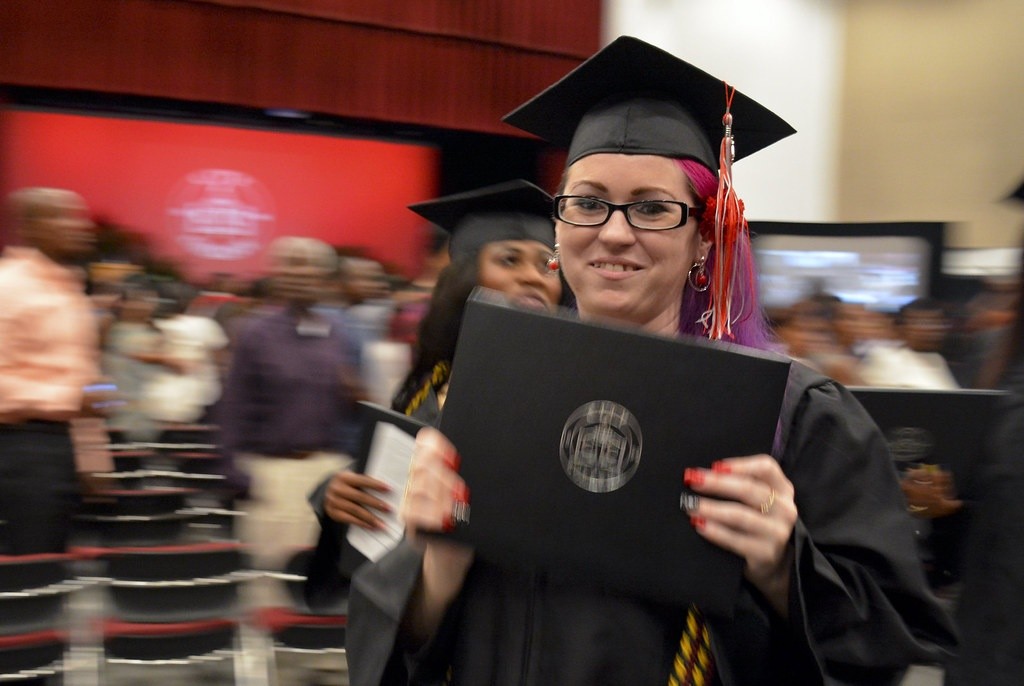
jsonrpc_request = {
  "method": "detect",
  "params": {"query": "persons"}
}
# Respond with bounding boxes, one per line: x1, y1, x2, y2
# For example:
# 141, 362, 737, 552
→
0, 179, 578, 686
347, 35, 927, 686
759, 259, 1024, 686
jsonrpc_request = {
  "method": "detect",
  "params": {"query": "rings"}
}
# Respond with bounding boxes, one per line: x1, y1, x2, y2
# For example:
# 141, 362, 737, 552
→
760, 488, 777, 514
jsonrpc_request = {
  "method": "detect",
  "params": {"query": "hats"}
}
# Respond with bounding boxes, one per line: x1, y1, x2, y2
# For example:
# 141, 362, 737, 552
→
501, 34, 797, 340
407, 180, 556, 274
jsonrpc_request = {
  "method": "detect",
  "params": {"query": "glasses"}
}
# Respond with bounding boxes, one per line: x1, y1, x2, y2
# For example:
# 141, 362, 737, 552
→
554, 195, 706, 231
